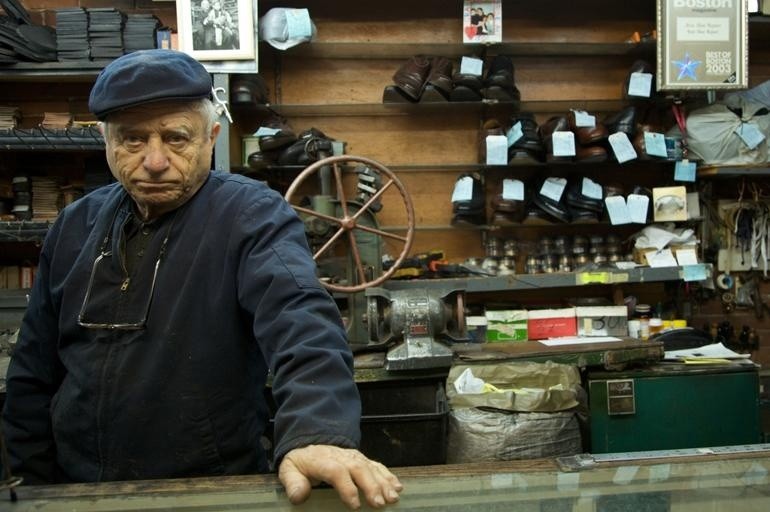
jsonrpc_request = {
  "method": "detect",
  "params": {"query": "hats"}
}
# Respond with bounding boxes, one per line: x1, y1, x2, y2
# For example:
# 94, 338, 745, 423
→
89, 48, 213, 121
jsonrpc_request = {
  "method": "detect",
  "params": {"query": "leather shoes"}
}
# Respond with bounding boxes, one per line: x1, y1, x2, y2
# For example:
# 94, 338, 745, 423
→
476, 107, 656, 164
450, 171, 655, 227
232, 76, 269, 106
246, 127, 337, 169
381, 53, 522, 104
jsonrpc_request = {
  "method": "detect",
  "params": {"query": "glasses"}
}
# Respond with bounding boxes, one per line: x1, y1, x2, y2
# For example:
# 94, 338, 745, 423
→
78, 249, 161, 336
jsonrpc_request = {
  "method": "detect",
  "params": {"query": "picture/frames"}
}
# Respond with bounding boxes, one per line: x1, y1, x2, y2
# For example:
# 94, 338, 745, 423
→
654, 0, 751, 95
460, 1, 504, 48
173, 0, 258, 63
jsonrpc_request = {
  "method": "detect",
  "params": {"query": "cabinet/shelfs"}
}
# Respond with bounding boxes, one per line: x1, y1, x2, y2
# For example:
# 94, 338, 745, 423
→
221, 0, 770, 300
0, 1, 221, 394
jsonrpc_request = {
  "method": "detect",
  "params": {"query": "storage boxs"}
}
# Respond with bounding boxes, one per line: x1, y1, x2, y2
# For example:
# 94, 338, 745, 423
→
578, 354, 764, 451
468, 307, 631, 342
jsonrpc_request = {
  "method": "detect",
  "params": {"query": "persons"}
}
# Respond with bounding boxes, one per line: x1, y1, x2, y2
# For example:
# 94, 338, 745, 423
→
1, 46, 405, 510
470, 6, 496, 35
202, 1, 233, 49
190, 0, 240, 49
